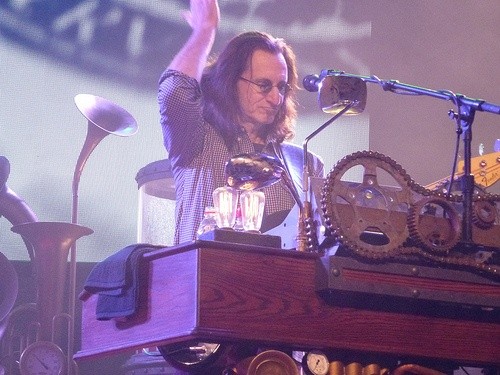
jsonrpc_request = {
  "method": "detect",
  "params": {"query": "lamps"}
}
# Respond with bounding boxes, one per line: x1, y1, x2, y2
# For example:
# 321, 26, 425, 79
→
295, 76, 369, 254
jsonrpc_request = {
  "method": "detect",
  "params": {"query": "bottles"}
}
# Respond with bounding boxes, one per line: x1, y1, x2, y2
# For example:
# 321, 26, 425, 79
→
197, 206, 219, 239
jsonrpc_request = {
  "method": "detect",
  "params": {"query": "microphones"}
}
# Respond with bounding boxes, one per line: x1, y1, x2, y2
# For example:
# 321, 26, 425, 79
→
303, 72, 323, 91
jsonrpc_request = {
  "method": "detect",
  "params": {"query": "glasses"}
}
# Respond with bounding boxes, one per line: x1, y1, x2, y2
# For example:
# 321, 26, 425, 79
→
239, 75, 292, 94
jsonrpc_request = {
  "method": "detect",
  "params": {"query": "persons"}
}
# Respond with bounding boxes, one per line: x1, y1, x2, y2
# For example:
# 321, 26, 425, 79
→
156, 0, 323, 245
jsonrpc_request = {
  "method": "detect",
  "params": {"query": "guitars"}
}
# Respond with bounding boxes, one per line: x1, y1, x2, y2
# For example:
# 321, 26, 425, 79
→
419, 137, 500, 192
